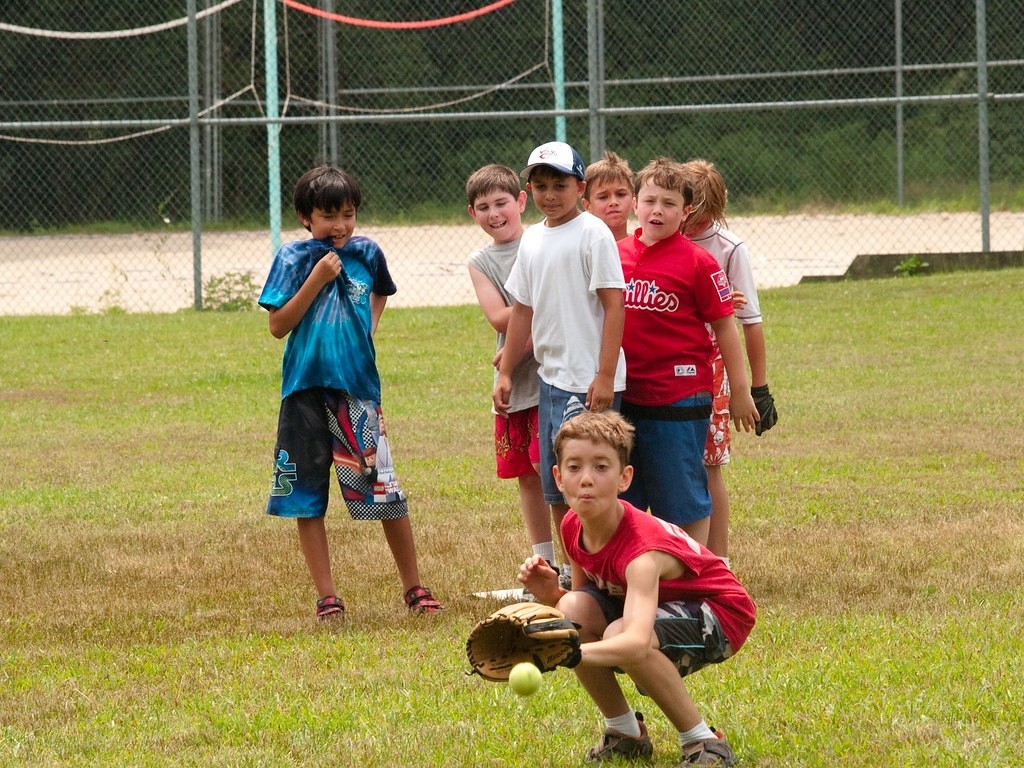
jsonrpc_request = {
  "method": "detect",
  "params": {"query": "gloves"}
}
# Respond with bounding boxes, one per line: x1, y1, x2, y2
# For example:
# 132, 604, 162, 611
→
750, 384, 778, 436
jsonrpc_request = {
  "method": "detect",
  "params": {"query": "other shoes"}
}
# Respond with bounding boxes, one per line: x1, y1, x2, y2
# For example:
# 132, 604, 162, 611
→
404, 586, 445, 613
521, 559, 560, 600
558, 569, 572, 592
316, 595, 345, 625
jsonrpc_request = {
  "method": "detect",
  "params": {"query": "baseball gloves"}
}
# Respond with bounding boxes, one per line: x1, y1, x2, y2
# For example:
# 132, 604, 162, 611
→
463, 602, 582, 682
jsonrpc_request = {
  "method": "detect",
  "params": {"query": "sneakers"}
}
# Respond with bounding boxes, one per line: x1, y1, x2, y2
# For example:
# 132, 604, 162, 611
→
586, 712, 653, 766
676, 726, 739, 768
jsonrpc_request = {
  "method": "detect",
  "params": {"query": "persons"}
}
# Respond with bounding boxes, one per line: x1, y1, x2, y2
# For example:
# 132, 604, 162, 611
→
579, 149, 636, 244
491, 142, 626, 591
677, 159, 780, 573
518, 409, 756, 768
258, 163, 444, 616
615, 155, 762, 548
464, 163, 572, 603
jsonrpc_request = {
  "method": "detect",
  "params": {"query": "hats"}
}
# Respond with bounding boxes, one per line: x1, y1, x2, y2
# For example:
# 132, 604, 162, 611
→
519, 141, 586, 181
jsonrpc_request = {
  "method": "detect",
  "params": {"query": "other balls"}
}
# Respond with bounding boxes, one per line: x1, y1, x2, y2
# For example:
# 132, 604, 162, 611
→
508, 661, 541, 695
